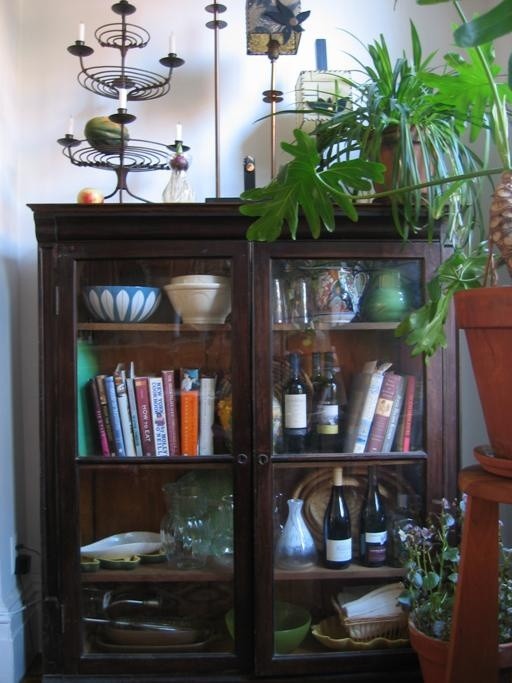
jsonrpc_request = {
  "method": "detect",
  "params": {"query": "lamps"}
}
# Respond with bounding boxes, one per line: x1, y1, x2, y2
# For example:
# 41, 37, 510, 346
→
57, 2, 190, 204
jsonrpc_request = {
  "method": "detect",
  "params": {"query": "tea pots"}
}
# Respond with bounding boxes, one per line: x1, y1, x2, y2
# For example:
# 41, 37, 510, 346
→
298, 257, 367, 325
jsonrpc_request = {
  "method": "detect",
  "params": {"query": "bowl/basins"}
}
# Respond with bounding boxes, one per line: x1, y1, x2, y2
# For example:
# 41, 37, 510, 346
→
225, 598, 311, 657
164, 273, 230, 324
82, 285, 161, 324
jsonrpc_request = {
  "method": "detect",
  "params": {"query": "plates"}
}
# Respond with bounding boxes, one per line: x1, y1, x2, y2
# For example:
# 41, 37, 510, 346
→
313, 618, 408, 649
92, 626, 222, 654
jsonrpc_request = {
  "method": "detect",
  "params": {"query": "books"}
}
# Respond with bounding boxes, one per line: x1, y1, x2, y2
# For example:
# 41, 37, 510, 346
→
341, 359, 425, 455
88, 362, 234, 456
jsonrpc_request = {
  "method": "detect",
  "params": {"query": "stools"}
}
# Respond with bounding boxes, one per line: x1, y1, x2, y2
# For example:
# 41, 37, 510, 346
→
447, 467, 511, 680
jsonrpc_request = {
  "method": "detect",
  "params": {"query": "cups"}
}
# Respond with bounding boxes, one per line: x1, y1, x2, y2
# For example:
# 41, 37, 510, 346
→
271, 277, 286, 324
289, 279, 313, 324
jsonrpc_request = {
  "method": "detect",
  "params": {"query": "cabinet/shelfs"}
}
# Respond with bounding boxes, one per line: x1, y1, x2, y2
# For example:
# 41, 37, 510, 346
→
29, 196, 460, 677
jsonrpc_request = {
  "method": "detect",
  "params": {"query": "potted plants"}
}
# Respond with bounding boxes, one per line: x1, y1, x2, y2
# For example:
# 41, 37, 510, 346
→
397, 495, 511, 682
237, 0, 512, 478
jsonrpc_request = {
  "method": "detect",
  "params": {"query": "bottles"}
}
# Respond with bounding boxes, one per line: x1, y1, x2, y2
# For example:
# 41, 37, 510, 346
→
361, 464, 386, 567
315, 354, 340, 453
276, 499, 317, 571
309, 353, 322, 407
323, 468, 353, 569
284, 355, 310, 453
390, 496, 420, 568
